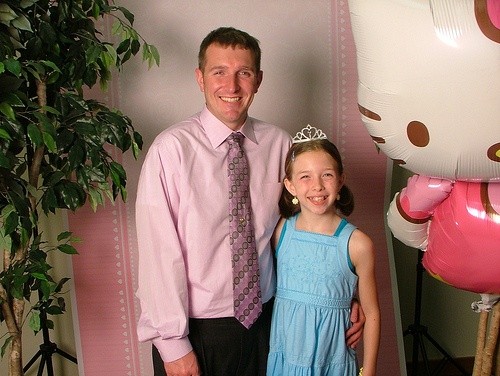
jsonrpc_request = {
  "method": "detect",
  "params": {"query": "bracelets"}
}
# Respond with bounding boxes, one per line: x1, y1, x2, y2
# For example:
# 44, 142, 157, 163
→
359, 367, 363, 376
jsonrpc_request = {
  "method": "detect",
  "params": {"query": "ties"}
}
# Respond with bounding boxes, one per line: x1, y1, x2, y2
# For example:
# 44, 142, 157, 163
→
224, 131, 263, 329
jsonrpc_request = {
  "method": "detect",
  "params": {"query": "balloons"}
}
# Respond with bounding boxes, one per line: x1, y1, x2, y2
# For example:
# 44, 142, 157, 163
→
349, 0, 500, 295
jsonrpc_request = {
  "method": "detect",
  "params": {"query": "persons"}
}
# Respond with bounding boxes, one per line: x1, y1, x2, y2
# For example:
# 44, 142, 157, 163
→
135, 27, 366, 376
266, 123, 381, 376
